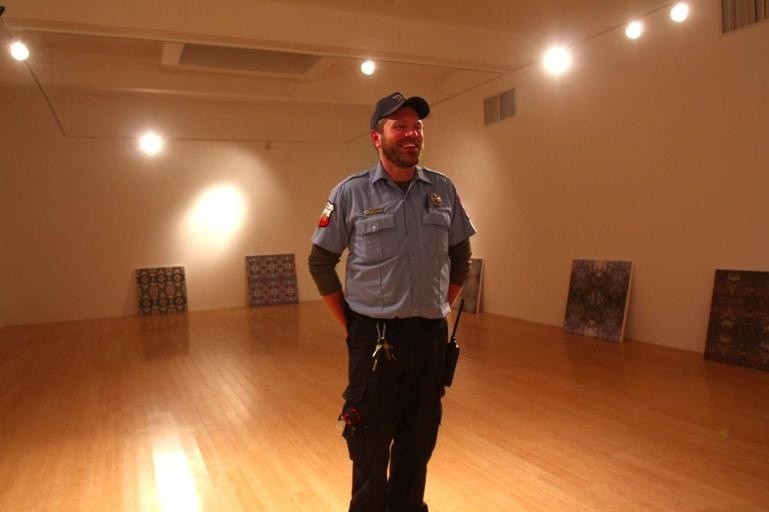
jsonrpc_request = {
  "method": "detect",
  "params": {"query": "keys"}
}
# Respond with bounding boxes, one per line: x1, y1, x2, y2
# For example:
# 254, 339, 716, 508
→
371, 336, 398, 372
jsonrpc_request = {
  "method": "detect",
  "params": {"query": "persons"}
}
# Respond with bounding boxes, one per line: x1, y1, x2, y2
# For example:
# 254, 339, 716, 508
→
306, 90, 479, 512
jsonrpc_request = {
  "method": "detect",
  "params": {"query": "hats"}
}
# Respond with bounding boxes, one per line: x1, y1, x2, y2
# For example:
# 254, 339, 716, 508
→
367, 91, 433, 130
